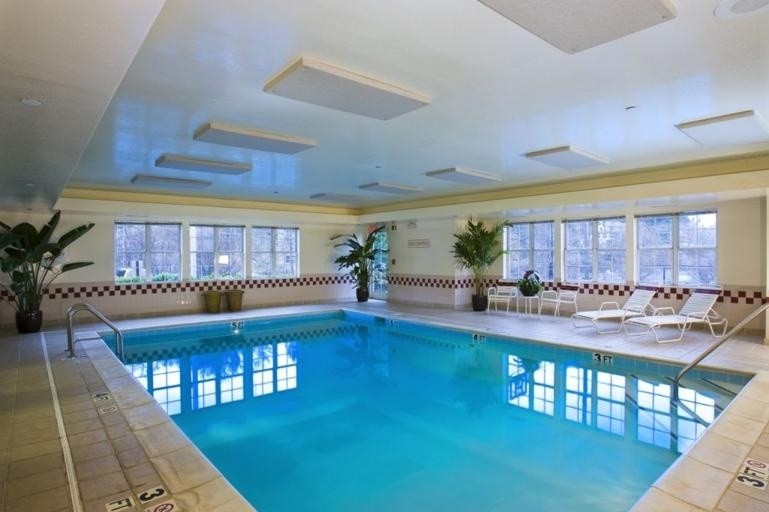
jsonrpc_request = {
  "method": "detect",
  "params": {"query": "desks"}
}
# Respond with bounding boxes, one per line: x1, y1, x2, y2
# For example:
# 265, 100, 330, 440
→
516, 295, 539, 314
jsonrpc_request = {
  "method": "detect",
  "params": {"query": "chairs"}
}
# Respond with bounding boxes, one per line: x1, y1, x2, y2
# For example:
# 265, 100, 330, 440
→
538, 282, 581, 317
623, 284, 728, 344
571, 281, 661, 335
487, 279, 518, 312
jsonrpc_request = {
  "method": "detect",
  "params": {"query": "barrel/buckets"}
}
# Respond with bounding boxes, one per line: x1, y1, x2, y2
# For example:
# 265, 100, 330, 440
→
201, 290, 222, 313
224, 290, 245, 312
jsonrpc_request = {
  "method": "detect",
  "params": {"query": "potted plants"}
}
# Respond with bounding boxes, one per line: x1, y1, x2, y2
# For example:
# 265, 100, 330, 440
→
449, 219, 513, 311
0, 209, 95, 334
331, 225, 388, 301
